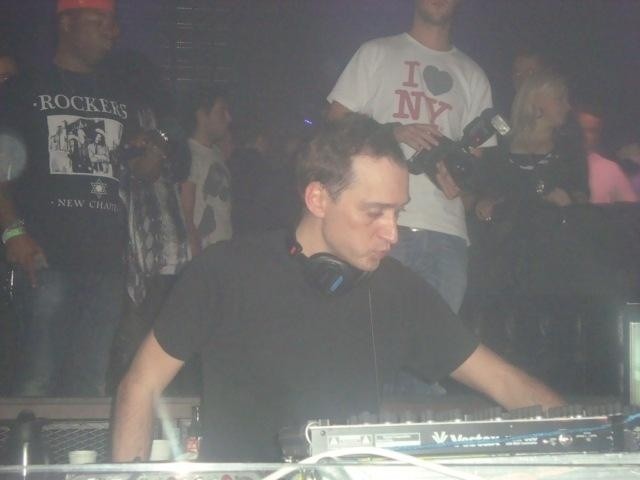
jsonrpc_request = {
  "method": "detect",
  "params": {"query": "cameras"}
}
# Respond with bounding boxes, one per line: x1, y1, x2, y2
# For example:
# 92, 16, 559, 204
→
405, 107, 512, 195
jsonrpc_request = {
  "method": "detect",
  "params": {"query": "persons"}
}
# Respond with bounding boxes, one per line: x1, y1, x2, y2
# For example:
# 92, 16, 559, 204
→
325, 0, 493, 312
0, 0, 194, 407
111, 113, 568, 465
505, 45, 640, 206
112, 79, 316, 389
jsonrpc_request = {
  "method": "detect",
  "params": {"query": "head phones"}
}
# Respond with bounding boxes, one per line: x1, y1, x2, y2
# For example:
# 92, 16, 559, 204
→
286, 208, 371, 294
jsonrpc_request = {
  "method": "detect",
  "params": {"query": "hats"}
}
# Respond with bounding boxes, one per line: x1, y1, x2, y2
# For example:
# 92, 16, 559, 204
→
55, 0, 117, 13
